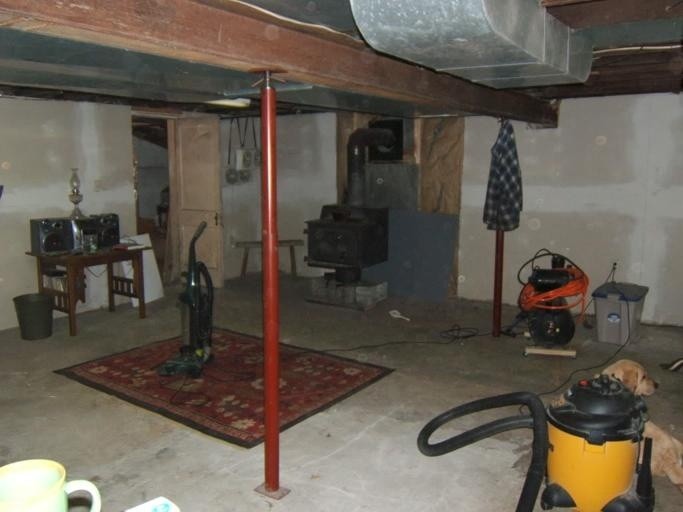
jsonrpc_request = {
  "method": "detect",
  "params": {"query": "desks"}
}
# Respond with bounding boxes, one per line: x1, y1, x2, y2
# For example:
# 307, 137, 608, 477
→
24, 244, 154, 337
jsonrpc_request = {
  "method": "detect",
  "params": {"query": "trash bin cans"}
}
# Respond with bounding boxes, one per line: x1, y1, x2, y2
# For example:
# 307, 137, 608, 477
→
592, 281, 649, 343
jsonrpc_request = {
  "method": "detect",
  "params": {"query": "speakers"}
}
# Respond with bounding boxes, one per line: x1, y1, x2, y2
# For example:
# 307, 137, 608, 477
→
30, 217, 74, 255
90, 213, 120, 249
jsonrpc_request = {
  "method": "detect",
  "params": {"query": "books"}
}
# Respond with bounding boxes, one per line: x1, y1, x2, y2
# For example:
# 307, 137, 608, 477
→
113, 242, 145, 250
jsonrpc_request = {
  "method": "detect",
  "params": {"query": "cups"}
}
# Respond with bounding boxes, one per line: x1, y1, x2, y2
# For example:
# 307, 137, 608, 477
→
0, 458, 102, 512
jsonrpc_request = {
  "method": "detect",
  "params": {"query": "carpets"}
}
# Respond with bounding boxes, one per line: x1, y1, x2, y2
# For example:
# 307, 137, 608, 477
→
51, 326, 397, 449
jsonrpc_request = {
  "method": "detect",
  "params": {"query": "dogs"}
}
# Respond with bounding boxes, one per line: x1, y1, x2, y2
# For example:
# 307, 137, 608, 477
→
593, 359, 683, 492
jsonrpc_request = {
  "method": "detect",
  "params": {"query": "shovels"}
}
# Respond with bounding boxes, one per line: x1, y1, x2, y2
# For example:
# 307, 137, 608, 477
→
389, 309, 410, 321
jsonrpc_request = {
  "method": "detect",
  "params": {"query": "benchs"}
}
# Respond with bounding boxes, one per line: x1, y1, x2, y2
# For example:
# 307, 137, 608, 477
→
234, 239, 304, 280
